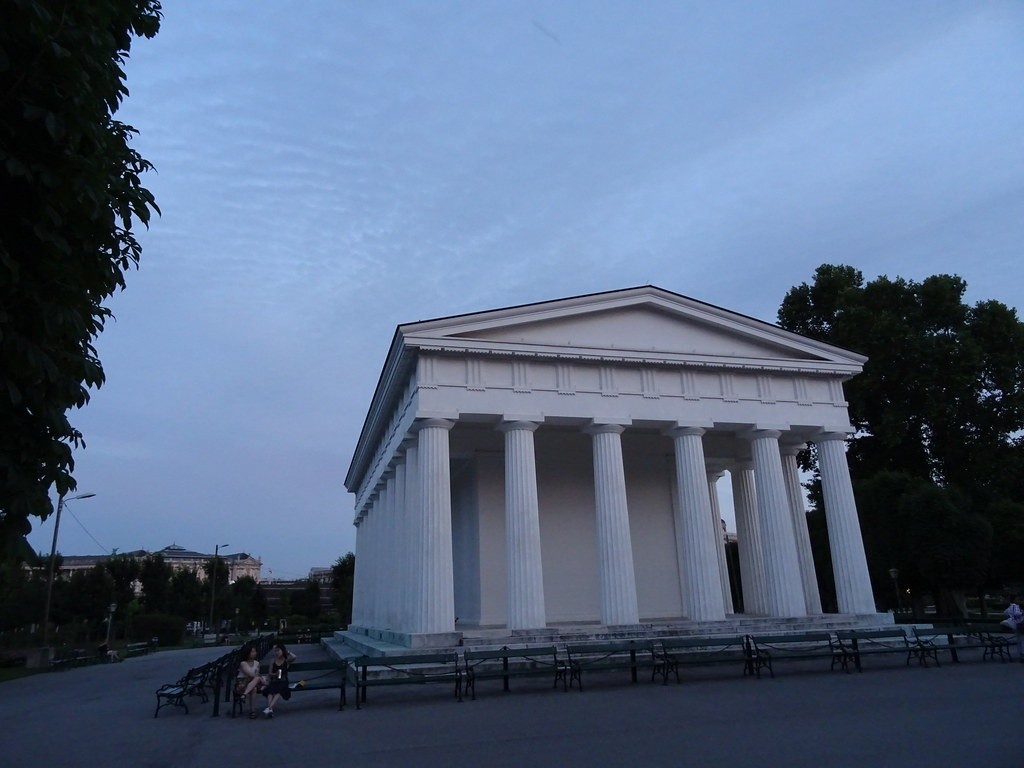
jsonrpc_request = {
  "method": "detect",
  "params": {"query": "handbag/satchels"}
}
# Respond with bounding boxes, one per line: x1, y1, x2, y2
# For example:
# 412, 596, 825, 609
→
1000, 618, 1016, 630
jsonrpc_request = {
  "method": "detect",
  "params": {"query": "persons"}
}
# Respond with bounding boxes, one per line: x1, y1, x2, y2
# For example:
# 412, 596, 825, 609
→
1004, 593, 1024, 663
238, 645, 265, 719
263, 644, 296, 718
98, 641, 124, 663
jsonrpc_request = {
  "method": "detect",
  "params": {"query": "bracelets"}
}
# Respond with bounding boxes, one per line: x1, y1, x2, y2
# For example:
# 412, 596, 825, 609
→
288, 651, 290, 655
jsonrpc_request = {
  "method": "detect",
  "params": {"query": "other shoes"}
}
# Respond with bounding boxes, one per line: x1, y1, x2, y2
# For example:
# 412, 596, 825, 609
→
239, 694, 247, 701
1019, 656, 1024, 660
262, 708, 273, 718
249, 711, 256, 719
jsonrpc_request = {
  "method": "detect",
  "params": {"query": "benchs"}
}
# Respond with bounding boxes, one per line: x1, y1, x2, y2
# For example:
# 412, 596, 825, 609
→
464, 647, 568, 700
660, 637, 761, 684
126, 641, 149, 657
912, 622, 1018, 667
230, 659, 347, 719
96, 640, 120, 664
835, 630, 930, 673
155, 633, 275, 719
226, 634, 242, 644
567, 642, 667, 691
353, 654, 464, 710
47, 642, 95, 673
752, 633, 850, 678
277, 630, 341, 645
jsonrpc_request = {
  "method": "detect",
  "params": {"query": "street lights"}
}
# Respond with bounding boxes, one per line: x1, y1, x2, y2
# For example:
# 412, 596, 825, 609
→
208, 544, 230, 633
40, 492, 96, 647
